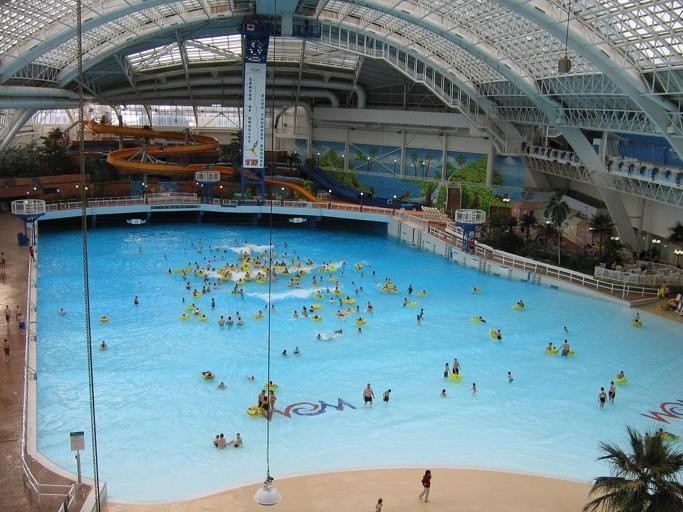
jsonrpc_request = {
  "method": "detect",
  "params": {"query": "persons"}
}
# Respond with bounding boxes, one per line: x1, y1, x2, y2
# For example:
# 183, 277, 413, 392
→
0, 338, 10, 361
3, 304, 11, 325
418, 469, 430, 503
14, 304, 21, 317
375, 498, 383, 511
99, 315, 108, 322
99, 340, 108, 349
128, 230, 683, 453
16, 312, 25, 334
58, 307, 66, 315
0, 252, 7, 272
27, 243, 35, 261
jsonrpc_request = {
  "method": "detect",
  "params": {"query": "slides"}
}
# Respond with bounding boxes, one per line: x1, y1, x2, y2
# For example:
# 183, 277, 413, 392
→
204, 162, 427, 210
88, 120, 320, 202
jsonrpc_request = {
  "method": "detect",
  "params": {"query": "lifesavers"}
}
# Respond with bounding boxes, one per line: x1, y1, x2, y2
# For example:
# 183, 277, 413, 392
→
449, 375, 462, 380
100, 317, 108, 321
568, 349, 574, 356
512, 305, 526, 310
168, 240, 427, 326
546, 350, 558, 353
489, 329, 497, 338
473, 317, 482, 322
264, 386, 278, 390
616, 377, 627, 383
247, 406, 263, 416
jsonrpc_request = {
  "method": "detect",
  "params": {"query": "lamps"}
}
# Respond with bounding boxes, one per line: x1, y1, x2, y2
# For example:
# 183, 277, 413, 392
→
254, 0, 282, 506
558, 0, 571, 73
